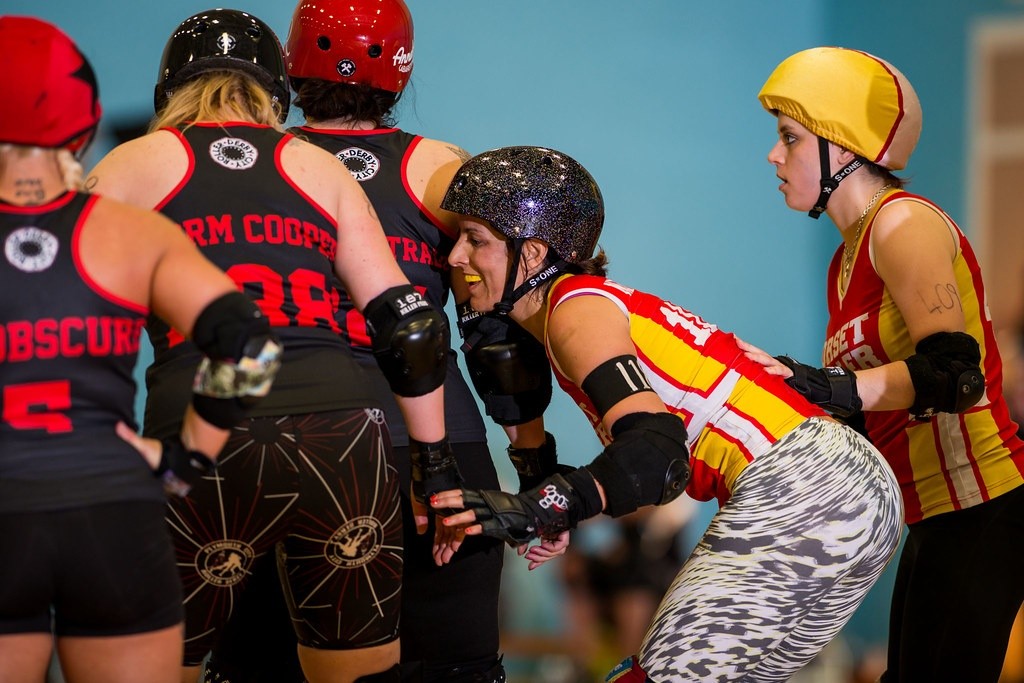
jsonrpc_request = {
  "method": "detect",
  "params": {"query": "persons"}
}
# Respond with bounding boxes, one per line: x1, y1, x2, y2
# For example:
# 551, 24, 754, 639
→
429, 147, 904, 683
79, 9, 472, 683
732, 45, 1024, 683
284, 0, 570, 683
554, 305, 1024, 683
0, 15, 282, 682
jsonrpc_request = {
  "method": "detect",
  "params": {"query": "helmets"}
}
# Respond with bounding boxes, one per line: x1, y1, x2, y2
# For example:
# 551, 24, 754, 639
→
154, 8, 291, 125
0, 15, 102, 153
758, 47, 923, 171
440, 145, 605, 274
284, 0, 414, 93
